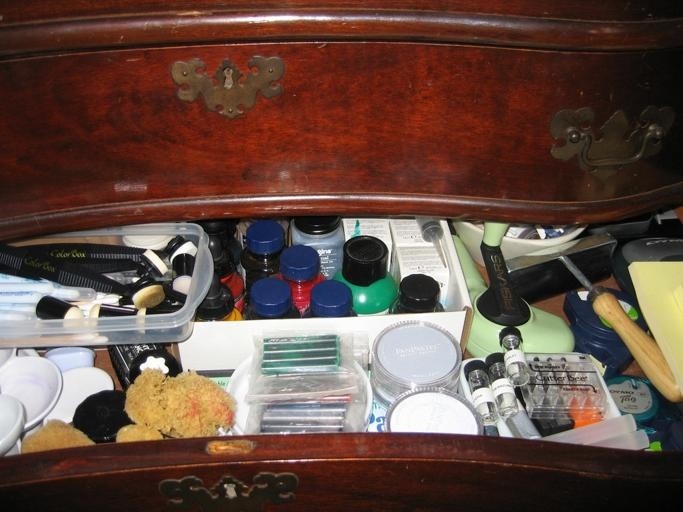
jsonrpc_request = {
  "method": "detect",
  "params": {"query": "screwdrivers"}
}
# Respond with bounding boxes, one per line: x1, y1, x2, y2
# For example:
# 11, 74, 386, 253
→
559, 255, 683, 404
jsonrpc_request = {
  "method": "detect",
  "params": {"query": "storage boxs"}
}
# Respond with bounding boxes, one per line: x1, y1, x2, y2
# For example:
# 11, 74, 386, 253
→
0, 221, 215, 349
163, 212, 474, 373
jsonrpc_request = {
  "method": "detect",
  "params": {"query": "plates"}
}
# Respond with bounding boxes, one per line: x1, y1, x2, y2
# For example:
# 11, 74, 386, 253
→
0, 346, 115, 455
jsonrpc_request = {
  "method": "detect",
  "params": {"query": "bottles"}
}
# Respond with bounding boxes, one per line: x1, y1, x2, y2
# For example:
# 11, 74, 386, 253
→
485, 351, 520, 418
498, 326, 531, 386
202, 216, 445, 324
461, 358, 499, 429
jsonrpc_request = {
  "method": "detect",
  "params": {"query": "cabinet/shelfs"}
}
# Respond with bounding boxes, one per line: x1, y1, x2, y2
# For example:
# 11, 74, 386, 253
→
0, 0, 681, 511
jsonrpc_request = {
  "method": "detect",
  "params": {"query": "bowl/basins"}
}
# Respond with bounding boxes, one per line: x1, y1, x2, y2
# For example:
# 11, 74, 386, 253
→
455, 219, 588, 268
